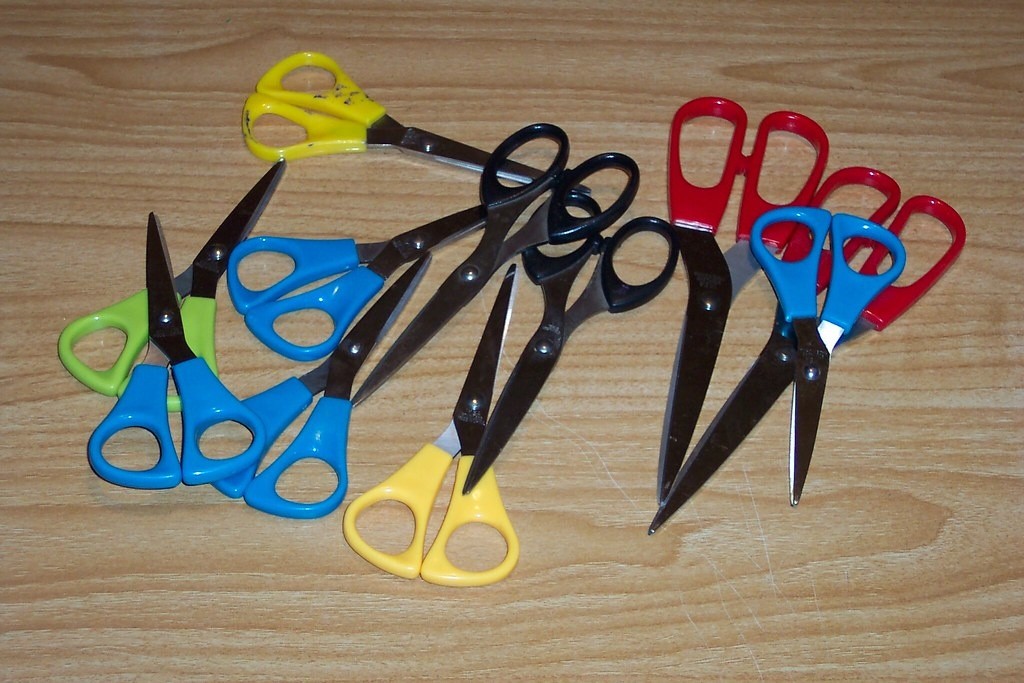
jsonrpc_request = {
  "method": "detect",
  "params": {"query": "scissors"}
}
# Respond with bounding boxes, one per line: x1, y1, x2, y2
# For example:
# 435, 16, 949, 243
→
53, 46, 679, 591
647, 94, 966, 539
